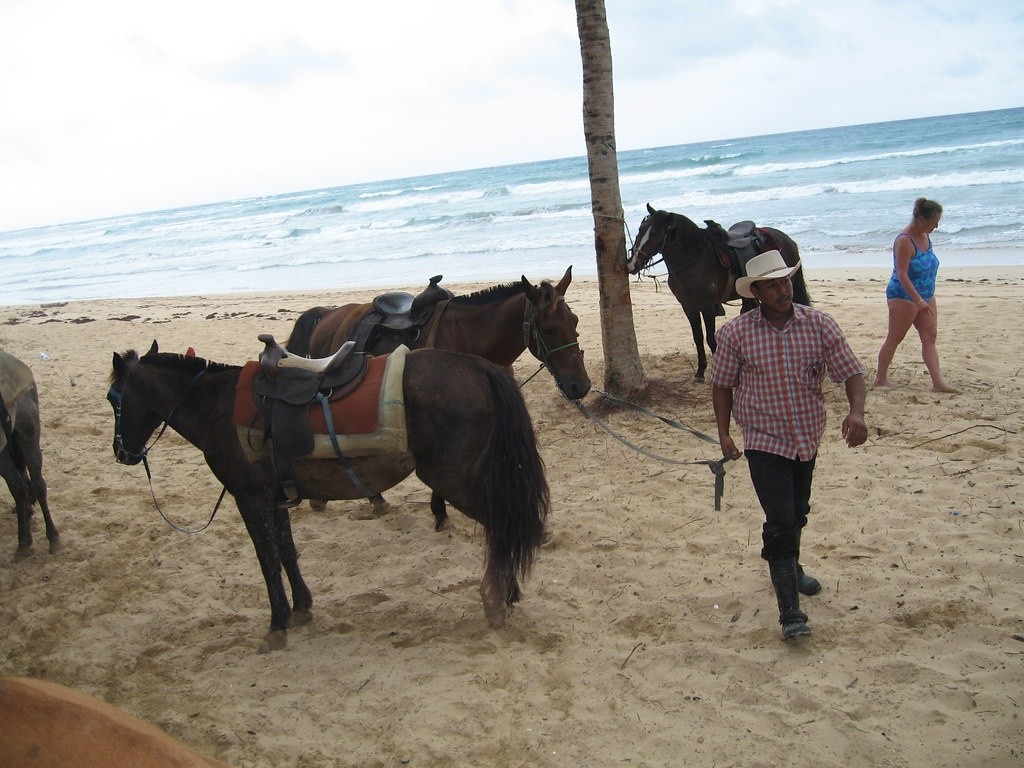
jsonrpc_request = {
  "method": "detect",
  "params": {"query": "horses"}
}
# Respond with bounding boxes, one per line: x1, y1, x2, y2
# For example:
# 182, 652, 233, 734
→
277, 263, 592, 536
625, 202, 812, 385
105, 336, 552, 658
0, 349, 66, 566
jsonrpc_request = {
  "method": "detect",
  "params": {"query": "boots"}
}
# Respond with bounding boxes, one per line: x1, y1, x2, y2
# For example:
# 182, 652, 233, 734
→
769, 556, 812, 641
796, 563, 821, 595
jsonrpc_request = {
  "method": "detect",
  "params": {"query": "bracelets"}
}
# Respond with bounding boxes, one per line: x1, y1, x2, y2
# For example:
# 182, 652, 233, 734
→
916, 299, 924, 305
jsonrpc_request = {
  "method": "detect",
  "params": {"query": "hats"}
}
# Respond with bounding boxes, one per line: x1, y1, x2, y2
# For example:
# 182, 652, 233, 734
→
735, 250, 801, 298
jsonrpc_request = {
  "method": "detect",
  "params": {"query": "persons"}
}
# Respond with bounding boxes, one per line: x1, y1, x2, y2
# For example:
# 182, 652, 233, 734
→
710, 250, 868, 640
872, 199, 962, 395
